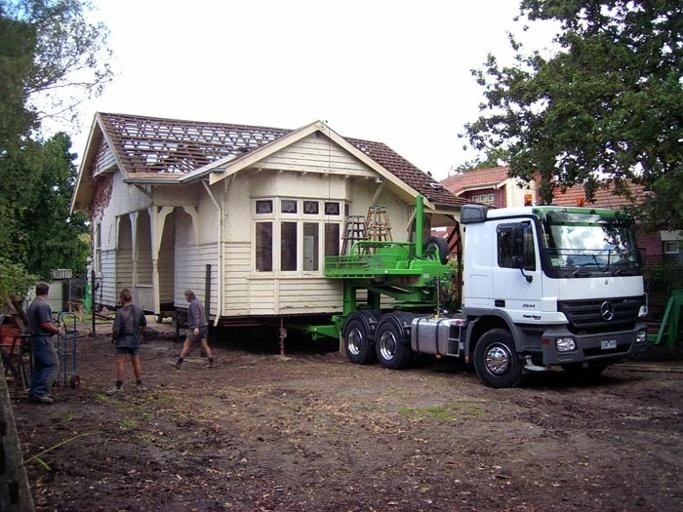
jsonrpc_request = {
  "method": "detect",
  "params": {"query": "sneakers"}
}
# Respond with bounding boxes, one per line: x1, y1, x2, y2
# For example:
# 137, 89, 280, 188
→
166, 360, 218, 370
28, 393, 54, 403
104, 385, 147, 394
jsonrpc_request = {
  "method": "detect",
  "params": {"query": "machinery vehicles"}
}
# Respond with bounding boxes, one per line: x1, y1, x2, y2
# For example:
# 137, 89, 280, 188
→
270, 193, 653, 390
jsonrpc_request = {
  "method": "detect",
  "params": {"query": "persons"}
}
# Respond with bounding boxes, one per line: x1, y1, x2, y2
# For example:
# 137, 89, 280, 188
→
104, 288, 147, 394
25, 284, 58, 405
166, 289, 217, 370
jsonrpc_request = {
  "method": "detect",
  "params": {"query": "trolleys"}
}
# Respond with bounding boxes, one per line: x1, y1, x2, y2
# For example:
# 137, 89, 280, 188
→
56, 310, 82, 389
